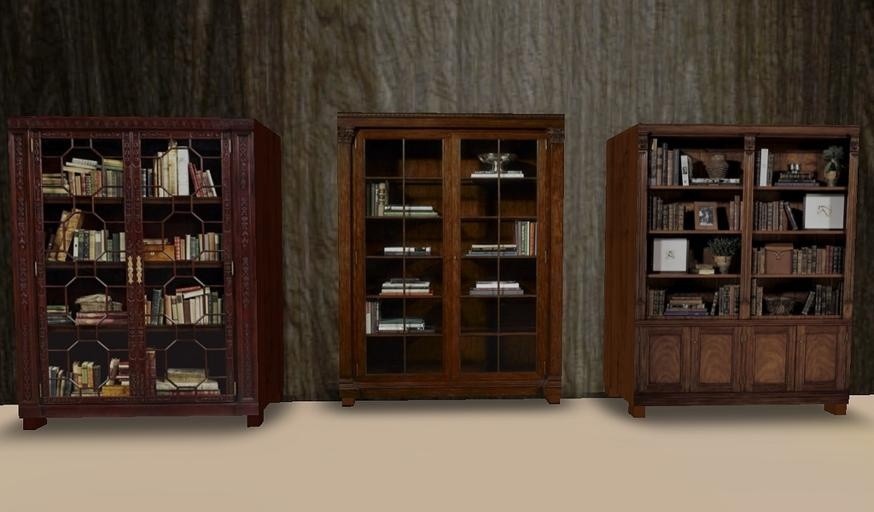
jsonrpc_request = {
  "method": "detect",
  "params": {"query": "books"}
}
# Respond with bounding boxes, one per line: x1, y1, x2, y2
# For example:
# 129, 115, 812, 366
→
47, 294, 128, 325
143, 285, 222, 324
647, 137, 843, 315
369, 164, 538, 340
142, 145, 217, 197
144, 232, 219, 260
41, 158, 123, 197
46, 209, 126, 262
147, 347, 221, 396
48, 358, 129, 396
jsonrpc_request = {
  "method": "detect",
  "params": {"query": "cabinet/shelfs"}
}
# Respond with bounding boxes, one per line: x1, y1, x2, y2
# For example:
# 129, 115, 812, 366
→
601, 114, 854, 423
7, 115, 283, 429
332, 110, 564, 407
